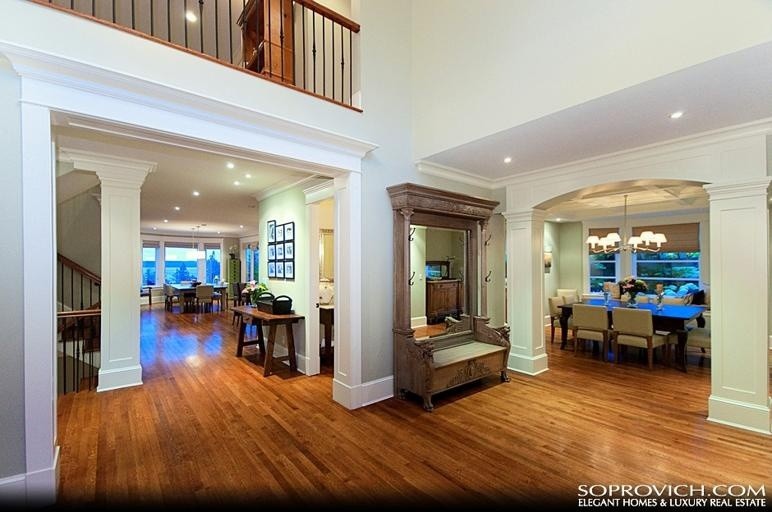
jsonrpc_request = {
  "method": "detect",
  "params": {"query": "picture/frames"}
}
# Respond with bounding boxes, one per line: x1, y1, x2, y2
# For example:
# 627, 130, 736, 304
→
266, 219, 296, 281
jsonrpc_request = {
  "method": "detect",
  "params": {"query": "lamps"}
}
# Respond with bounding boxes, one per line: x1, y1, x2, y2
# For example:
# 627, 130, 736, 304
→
190, 225, 203, 260
585, 195, 667, 255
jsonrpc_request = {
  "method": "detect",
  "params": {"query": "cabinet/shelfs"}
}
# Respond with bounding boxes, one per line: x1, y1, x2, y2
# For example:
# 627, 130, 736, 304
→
235, 0, 294, 88
427, 277, 461, 326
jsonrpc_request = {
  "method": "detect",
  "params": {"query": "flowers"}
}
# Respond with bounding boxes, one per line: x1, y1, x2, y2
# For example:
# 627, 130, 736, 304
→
242, 279, 269, 303
618, 273, 648, 299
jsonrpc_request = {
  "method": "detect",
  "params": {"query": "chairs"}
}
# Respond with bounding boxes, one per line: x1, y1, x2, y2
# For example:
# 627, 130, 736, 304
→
232, 281, 250, 327
548, 281, 708, 374
163, 279, 228, 314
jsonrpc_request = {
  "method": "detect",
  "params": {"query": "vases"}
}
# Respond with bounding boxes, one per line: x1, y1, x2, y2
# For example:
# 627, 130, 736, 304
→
250, 295, 259, 308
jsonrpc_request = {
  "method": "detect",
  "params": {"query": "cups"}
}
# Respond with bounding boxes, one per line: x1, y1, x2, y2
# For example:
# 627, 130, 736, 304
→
578, 295, 582, 302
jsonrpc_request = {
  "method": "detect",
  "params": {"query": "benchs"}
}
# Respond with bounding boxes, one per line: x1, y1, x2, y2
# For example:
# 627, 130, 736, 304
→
417, 339, 512, 412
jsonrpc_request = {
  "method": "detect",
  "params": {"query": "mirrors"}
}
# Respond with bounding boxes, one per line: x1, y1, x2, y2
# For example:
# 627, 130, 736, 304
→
384, 181, 513, 414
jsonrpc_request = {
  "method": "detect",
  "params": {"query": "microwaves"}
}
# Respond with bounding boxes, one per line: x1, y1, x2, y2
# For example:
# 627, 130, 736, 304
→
426, 260, 450, 280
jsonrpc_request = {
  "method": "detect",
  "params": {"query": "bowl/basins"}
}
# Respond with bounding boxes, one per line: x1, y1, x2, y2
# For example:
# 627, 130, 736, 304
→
191, 281, 201, 287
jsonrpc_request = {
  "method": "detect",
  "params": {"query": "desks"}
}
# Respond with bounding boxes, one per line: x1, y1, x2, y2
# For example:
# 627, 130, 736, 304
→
230, 304, 306, 377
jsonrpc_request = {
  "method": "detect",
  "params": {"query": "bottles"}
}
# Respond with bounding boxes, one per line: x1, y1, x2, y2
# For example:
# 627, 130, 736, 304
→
604, 288, 610, 306
